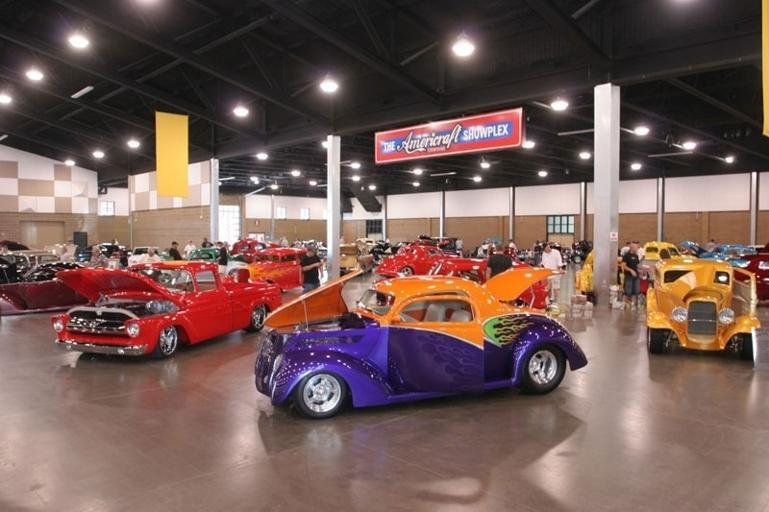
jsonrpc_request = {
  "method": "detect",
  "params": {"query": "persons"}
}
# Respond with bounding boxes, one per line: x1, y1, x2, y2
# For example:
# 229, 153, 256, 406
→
689, 240, 700, 257
299, 243, 324, 292
185, 235, 228, 265
708, 238, 722, 251
146, 246, 160, 263
619, 239, 646, 310
59, 246, 69, 262
108, 239, 119, 256
0, 241, 12, 257
92, 245, 108, 266
443, 234, 562, 303
168, 241, 183, 260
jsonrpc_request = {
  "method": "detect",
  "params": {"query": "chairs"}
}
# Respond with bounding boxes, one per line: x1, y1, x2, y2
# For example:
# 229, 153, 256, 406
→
423, 303, 472, 322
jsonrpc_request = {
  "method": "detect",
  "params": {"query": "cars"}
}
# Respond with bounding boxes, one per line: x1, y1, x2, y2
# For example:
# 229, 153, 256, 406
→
646, 258, 762, 359
254, 265, 588, 419
50, 259, 285, 360
